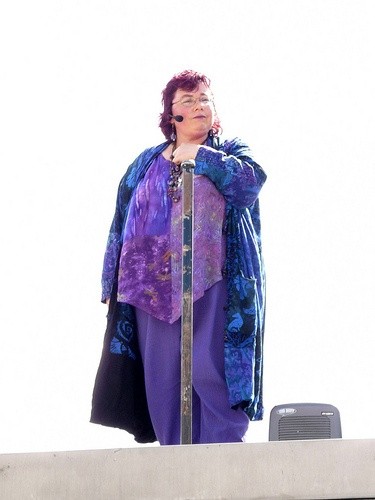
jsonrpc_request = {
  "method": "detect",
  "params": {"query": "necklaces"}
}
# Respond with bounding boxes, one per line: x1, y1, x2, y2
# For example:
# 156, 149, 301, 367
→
167, 138, 208, 203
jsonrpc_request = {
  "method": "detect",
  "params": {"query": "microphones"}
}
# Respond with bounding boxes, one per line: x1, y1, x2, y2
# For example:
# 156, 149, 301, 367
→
168, 114, 183, 122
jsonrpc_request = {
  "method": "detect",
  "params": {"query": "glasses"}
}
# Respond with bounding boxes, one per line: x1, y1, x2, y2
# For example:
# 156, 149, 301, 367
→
170, 95, 210, 106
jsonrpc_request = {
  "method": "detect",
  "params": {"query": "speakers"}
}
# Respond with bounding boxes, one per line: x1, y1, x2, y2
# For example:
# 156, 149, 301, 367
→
269, 404, 342, 441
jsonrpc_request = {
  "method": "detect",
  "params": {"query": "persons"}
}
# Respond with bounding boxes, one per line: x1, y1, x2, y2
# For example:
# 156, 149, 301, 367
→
89, 70, 268, 448
